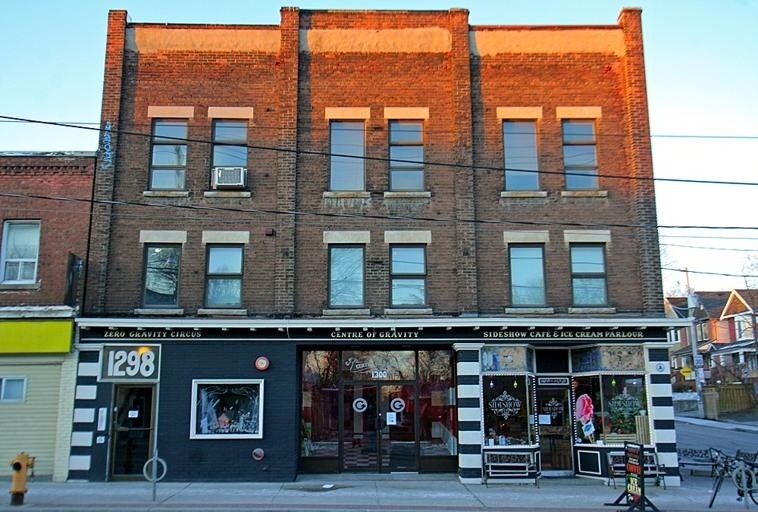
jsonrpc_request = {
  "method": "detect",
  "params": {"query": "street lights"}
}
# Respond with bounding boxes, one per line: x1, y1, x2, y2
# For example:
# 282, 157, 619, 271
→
686, 293, 705, 418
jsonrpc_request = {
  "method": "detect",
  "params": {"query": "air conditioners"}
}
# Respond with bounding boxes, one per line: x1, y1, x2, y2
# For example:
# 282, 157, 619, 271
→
210, 164, 247, 192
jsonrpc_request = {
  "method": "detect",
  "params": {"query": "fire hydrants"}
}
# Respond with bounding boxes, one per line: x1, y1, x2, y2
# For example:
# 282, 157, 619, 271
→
6, 449, 35, 507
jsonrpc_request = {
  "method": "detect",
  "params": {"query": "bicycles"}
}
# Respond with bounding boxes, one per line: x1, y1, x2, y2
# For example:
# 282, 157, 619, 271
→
705, 446, 758, 508
